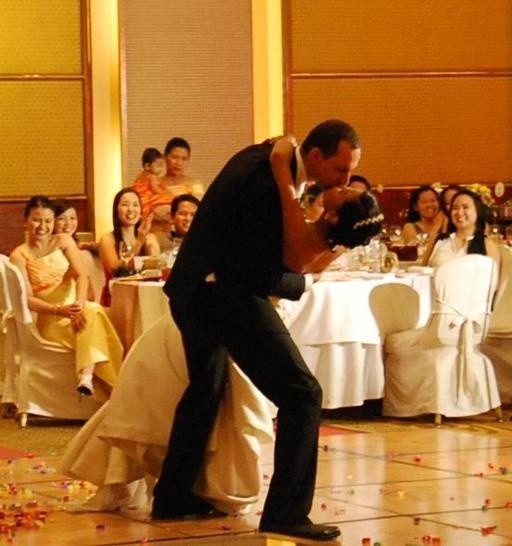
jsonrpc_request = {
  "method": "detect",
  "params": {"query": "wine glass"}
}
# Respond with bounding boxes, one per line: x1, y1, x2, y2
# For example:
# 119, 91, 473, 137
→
380, 224, 402, 244
119, 239, 135, 275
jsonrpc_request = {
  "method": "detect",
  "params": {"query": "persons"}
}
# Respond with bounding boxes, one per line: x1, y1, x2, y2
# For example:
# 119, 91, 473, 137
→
149, 119, 362, 542
60, 133, 384, 520
305, 185, 325, 222
348, 175, 502, 293
9, 136, 204, 398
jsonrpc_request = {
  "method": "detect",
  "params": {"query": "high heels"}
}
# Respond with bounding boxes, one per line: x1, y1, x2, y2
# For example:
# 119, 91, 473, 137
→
58, 485, 134, 513
77, 375, 94, 402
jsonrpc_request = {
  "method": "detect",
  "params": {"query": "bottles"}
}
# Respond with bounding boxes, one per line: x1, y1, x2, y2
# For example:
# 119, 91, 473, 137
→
415, 232, 431, 264
380, 241, 399, 274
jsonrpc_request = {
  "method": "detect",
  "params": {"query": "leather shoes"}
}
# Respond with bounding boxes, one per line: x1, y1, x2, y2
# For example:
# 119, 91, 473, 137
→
149, 502, 230, 519
257, 517, 342, 540
